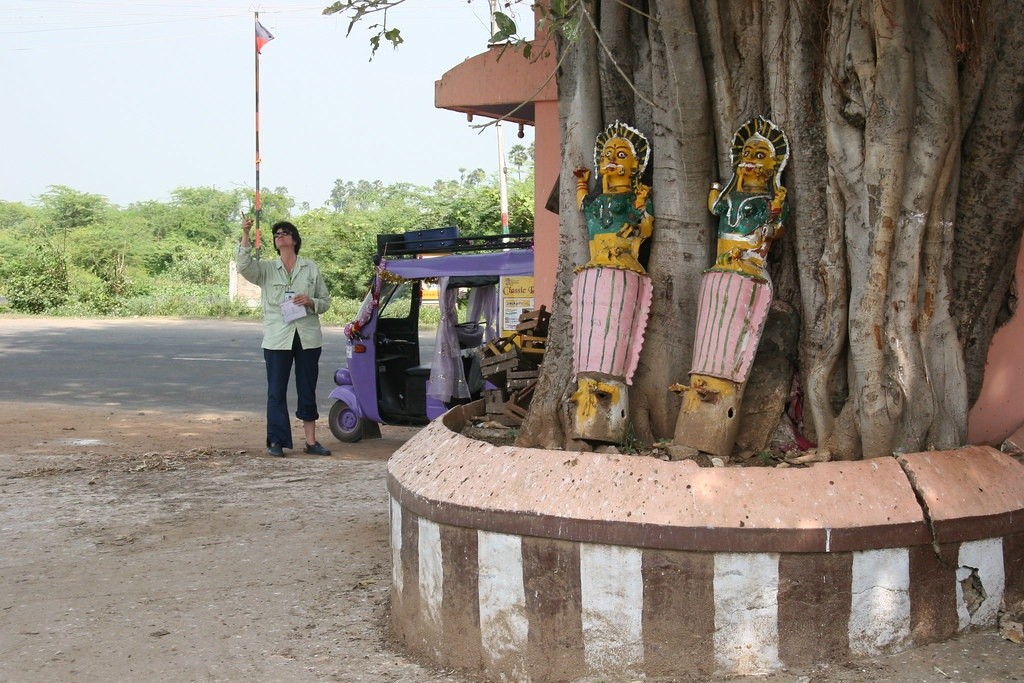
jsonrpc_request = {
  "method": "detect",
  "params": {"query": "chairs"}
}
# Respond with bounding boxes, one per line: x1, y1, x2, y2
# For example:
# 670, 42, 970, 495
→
401, 321, 485, 378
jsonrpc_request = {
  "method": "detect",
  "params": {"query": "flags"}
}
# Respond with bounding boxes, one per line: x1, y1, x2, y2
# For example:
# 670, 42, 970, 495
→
254, 17, 273, 54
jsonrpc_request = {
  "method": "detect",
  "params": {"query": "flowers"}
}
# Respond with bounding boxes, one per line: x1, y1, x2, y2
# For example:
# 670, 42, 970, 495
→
344, 321, 370, 345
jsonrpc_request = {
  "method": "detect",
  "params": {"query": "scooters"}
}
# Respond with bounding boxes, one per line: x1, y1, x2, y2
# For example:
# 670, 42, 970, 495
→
327, 226, 534, 443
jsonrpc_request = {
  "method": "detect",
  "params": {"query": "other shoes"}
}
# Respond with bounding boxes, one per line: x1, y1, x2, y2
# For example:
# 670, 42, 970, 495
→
304, 440, 331, 456
268, 445, 283, 457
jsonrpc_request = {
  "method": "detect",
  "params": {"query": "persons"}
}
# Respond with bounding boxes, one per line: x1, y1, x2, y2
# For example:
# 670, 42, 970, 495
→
689, 119, 791, 388
237, 210, 331, 455
568, 114, 661, 387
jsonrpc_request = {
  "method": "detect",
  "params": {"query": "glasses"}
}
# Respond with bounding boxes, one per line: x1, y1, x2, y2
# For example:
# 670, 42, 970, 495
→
273, 231, 293, 238
